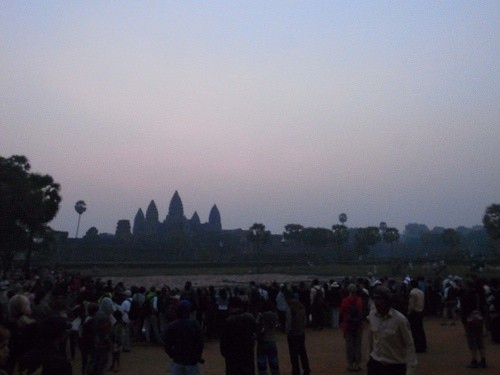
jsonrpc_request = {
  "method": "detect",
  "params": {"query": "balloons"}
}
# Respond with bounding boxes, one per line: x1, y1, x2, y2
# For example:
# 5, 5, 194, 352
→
75, 201, 88, 214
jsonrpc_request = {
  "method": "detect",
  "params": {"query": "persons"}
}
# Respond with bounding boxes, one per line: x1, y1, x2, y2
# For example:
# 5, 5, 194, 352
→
253, 301, 282, 375
219, 300, 259, 375
283, 294, 313, 375
407, 281, 428, 354
459, 279, 488, 369
163, 274, 499, 330
339, 285, 362, 370
164, 304, 204, 375
366, 283, 417, 375
1, 263, 163, 375
